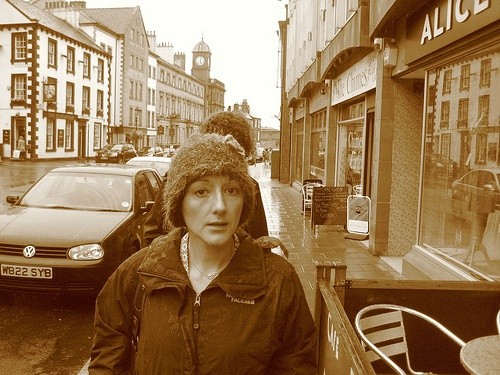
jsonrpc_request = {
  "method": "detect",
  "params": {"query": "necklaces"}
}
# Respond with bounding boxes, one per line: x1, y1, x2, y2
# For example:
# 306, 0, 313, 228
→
189, 256, 233, 280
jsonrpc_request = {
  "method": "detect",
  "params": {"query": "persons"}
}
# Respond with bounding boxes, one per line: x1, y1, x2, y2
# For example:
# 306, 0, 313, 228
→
263, 148, 269, 165
17, 136, 26, 160
251, 150, 257, 167
87, 132, 319, 375
142, 111, 271, 253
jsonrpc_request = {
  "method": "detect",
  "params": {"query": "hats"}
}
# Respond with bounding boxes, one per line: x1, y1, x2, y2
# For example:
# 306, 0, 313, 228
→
164, 132, 256, 229
202, 110, 254, 157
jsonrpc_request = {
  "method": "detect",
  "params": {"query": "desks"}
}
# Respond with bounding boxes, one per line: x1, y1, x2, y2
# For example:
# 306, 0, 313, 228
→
459, 334, 500, 375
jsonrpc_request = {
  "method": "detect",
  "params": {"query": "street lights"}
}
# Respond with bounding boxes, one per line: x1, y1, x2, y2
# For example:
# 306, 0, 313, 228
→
134, 105, 140, 151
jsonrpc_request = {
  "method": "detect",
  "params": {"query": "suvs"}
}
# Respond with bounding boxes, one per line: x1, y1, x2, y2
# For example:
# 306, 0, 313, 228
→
95, 143, 137, 164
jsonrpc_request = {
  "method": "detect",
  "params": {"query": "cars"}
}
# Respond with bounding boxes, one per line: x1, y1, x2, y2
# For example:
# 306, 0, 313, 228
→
450, 168, 500, 226
0, 162, 165, 295
108, 157, 172, 189
425, 153, 458, 179
137, 146, 176, 158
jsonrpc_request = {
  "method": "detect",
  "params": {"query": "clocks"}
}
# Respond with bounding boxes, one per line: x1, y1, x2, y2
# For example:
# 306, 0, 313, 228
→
196, 56, 205, 65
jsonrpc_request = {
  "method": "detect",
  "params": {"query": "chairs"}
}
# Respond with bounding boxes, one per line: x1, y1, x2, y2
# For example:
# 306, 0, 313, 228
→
302, 183, 324, 219
354, 303, 465, 375
